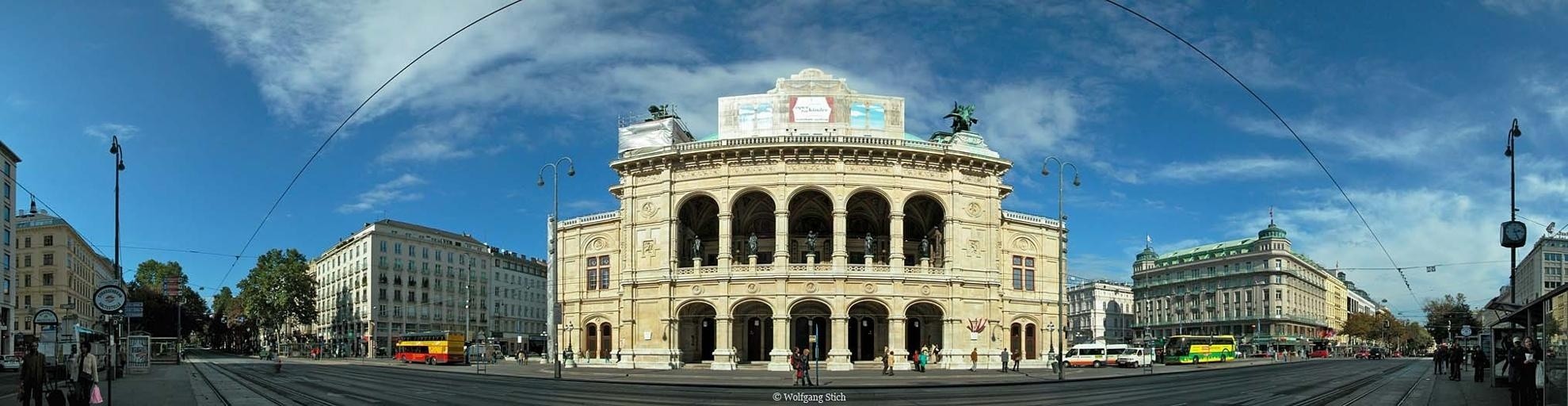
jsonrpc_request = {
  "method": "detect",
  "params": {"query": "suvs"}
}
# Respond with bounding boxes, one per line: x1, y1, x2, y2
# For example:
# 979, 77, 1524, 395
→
0, 354, 21, 372
1355, 349, 1369, 359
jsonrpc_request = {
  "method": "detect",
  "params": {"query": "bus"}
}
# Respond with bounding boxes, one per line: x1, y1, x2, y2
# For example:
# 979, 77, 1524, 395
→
1163, 333, 1236, 364
1452, 335, 1479, 352
395, 330, 465, 365
39, 326, 109, 379
1133, 338, 1167, 364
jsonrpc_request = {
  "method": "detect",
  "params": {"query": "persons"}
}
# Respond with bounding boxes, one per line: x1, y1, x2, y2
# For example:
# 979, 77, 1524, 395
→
264, 328, 399, 361
1433, 343, 1486, 383
691, 235, 702, 257
748, 232, 758, 254
19, 341, 126, 406
1508, 334, 1555, 405
481, 351, 496, 364
865, 232, 873, 255
913, 343, 941, 372
969, 348, 978, 372
1283, 348, 1288, 362
881, 350, 889, 375
515, 348, 527, 367
606, 348, 610, 363
1012, 349, 1020, 371
805, 230, 818, 254
1001, 348, 1009, 373
1156, 345, 1186, 363
792, 347, 813, 386
562, 347, 590, 367
922, 235, 929, 257
887, 351, 895, 375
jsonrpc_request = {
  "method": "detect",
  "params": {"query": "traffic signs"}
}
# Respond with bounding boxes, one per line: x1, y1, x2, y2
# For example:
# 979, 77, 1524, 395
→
123, 307, 144, 313
163, 289, 183, 297
123, 302, 144, 307
163, 283, 183, 290
60, 304, 75, 310
163, 275, 183, 283
123, 312, 145, 318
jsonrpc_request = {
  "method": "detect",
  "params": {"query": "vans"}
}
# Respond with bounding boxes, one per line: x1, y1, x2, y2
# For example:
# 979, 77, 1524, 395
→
1368, 347, 1386, 360
1117, 348, 1155, 367
464, 343, 504, 360
1062, 343, 1107, 367
1106, 344, 1128, 365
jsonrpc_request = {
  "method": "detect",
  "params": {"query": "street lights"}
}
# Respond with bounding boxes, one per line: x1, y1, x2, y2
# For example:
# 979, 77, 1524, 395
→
565, 320, 577, 368
1045, 320, 1056, 370
1504, 118, 1523, 330
539, 330, 550, 364
110, 135, 129, 378
460, 251, 471, 342
1042, 155, 1081, 380
536, 157, 577, 378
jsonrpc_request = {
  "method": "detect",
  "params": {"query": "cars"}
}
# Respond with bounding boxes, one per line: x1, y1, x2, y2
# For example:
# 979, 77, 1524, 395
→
1411, 352, 1434, 357
1308, 349, 1329, 358
1235, 342, 1276, 358
1391, 350, 1402, 358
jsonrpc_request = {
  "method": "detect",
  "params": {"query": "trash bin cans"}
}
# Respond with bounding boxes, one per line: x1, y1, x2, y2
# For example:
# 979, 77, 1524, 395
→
1052, 362, 1058, 372
